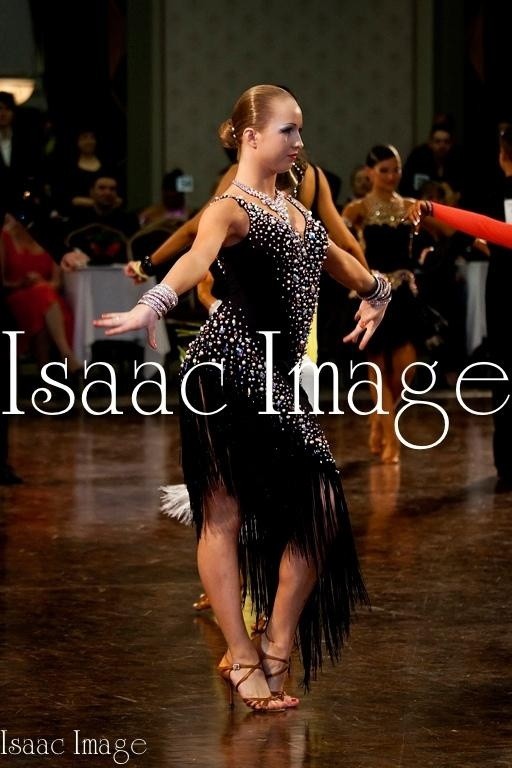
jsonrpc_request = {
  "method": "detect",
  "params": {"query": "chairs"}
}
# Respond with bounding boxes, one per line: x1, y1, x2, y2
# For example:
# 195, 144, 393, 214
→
58, 218, 198, 379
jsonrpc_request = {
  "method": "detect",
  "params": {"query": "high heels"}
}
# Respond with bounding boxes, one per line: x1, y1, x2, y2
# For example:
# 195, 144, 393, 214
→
365, 416, 402, 466
218, 635, 299, 713
189, 594, 214, 610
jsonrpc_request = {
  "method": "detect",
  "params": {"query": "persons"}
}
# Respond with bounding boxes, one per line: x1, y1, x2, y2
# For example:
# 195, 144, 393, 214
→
2, 84, 511, 712
